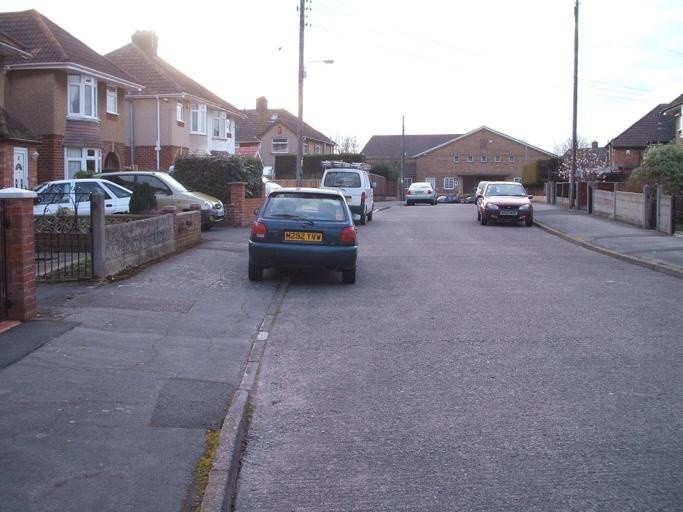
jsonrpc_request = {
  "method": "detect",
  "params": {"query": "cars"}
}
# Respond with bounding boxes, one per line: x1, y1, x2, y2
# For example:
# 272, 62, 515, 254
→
474, 181, 535, 227
29, 164, 281, 227
243, 183, 363, 288
404, 179, 488, 209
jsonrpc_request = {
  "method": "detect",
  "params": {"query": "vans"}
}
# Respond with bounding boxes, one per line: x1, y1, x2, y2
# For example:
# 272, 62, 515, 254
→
317, 160, 377, 225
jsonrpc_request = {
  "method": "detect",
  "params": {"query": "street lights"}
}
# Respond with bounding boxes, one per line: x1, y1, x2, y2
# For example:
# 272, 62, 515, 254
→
295, 59, 335, 185
